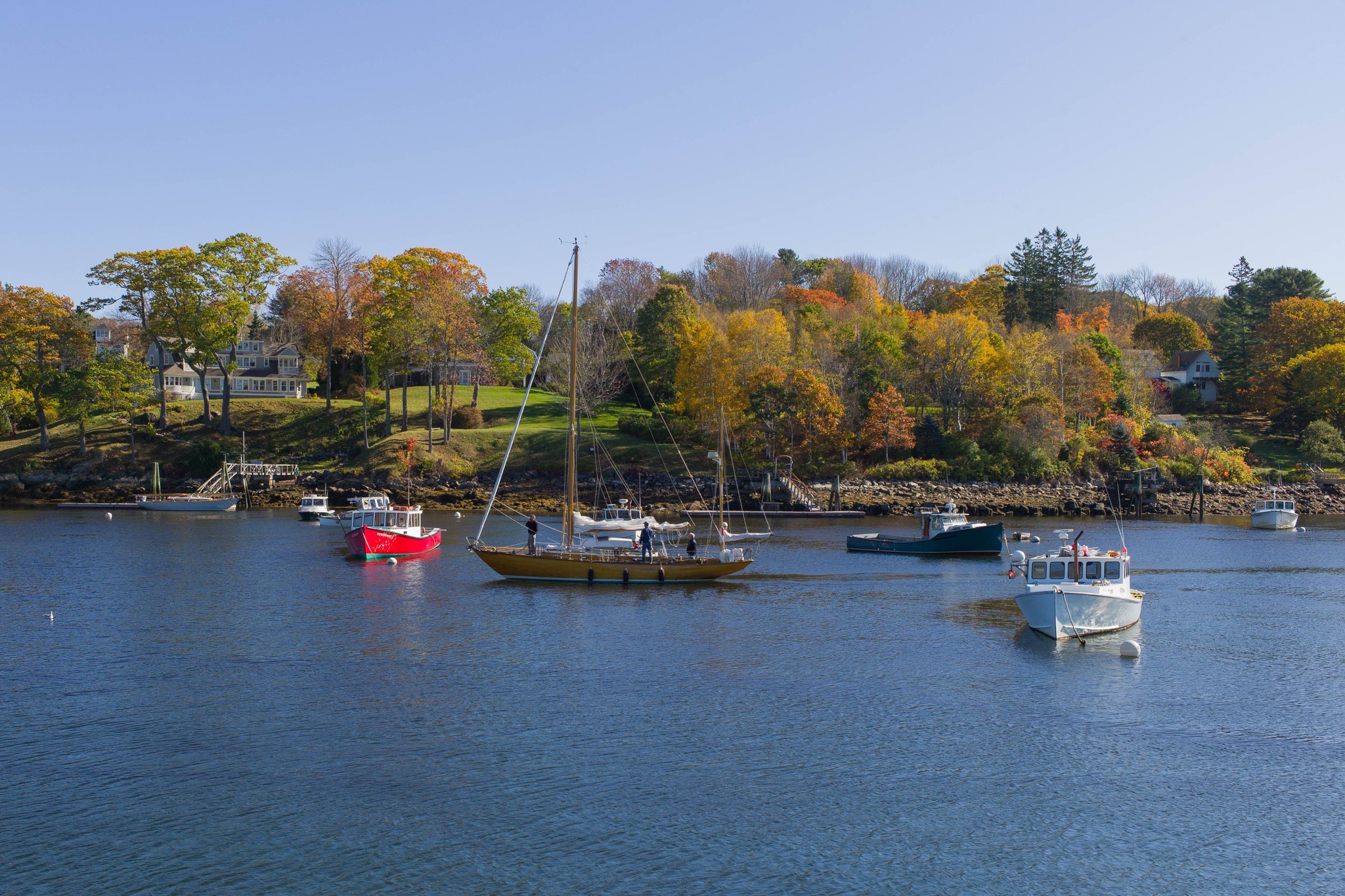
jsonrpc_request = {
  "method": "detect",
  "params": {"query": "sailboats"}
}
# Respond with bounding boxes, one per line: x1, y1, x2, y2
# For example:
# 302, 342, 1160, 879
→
465, 235, 778, 582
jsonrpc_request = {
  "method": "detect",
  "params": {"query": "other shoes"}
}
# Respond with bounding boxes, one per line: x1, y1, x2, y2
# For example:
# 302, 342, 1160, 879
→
526, 552, 531, 555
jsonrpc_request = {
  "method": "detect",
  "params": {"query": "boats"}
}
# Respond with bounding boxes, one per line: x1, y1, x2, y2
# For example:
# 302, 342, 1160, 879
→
317, 483, 422, 528
1247, 461, 1299, 530
132, 491, 246, 511
337, 465, 441, 561
1007, 481, 1146, 641
599, 472, 644, 543
298, 482, 335, 520
845, 466, 1005, 556
1012, 531, 1031, 541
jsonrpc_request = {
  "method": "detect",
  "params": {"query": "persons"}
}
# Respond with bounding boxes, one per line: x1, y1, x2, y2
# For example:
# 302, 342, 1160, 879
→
525, 514, 538, 556
686, 533, 697, 559
640, 521, 654, 562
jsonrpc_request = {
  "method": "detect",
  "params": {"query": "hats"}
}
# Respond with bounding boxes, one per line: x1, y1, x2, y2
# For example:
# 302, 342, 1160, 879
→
643, 521, 649, 524
